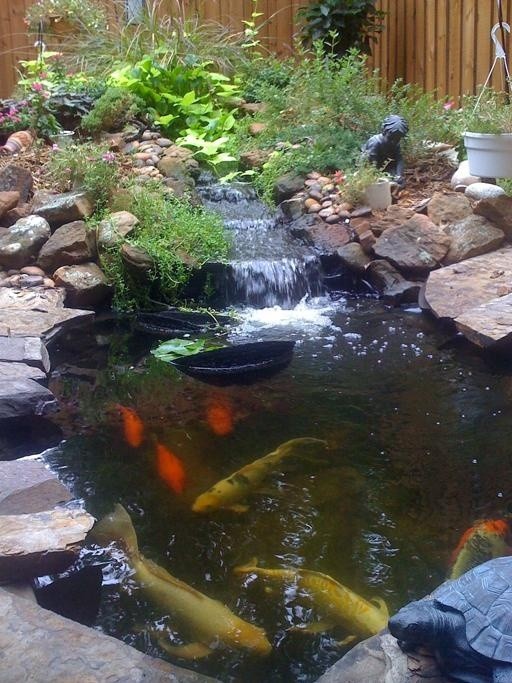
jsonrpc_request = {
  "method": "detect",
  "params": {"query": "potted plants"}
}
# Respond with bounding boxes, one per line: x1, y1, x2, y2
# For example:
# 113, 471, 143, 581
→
460, 23, 510, 181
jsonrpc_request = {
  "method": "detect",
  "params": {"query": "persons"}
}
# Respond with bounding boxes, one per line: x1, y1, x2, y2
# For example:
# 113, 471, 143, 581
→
357, 114, 410, 191
